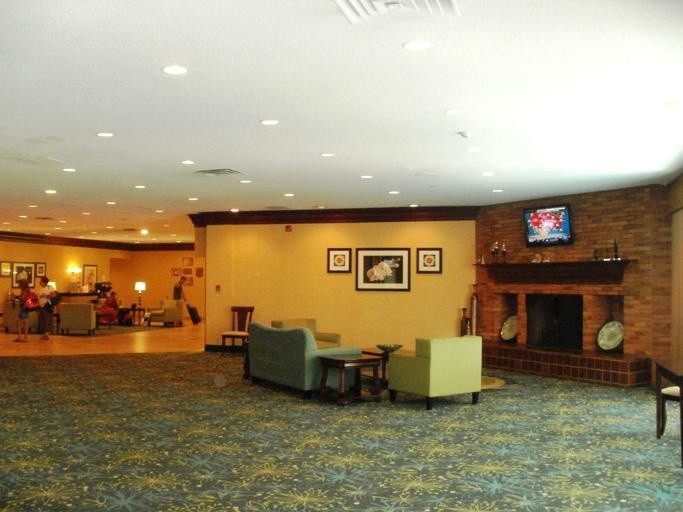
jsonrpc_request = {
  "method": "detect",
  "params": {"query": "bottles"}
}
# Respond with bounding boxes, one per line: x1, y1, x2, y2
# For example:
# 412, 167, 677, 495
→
8, 288, 11, 301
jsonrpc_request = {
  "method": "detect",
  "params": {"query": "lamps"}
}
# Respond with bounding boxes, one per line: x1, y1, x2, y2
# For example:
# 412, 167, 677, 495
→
134, 282, 146, 326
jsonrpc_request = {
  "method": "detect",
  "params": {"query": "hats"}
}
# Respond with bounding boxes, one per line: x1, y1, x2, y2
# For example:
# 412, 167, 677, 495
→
50, 294, 62, 305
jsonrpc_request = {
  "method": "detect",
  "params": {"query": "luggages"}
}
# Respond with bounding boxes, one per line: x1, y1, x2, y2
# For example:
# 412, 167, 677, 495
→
186, 303, 201, 324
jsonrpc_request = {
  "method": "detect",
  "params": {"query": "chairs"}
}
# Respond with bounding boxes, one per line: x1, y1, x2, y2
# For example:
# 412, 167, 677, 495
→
271, 318, 340, 348
388, 334, 483, 410
5, 301, 183, 337
655, 361, 683, 439
221, 306, 254, 356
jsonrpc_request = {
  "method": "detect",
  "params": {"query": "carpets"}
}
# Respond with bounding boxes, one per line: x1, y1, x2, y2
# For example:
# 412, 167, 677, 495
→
0, 350, 682, 511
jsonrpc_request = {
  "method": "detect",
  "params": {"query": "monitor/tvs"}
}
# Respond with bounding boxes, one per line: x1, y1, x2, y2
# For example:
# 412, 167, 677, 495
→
523, 203, 573, 248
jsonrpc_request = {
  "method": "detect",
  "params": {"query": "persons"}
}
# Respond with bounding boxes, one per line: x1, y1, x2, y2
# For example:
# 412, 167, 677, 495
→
11, 279, 33, 342
32, 276, 57, 341
173, 275, 187, 302
104, 291, 122, 309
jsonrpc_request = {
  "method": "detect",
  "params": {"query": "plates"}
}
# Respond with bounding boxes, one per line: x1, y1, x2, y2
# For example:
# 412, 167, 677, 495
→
596, 321, 623, 350
499, 315, 517, 340
377, 344, 402, 352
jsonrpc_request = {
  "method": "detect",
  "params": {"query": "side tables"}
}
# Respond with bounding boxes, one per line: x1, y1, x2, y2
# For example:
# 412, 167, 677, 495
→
319, 354, 383, 405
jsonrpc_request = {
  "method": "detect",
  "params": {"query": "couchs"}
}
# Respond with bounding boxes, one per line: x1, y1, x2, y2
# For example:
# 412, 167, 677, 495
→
248, 322, 361, 399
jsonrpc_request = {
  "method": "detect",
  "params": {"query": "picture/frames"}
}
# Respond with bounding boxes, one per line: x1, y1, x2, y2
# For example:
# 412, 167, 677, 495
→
327, 247, 352, 272
355, 248, 410, 292
83, 264, 97, 290
0, 261, 46, 288
417, 248, 442, 273
